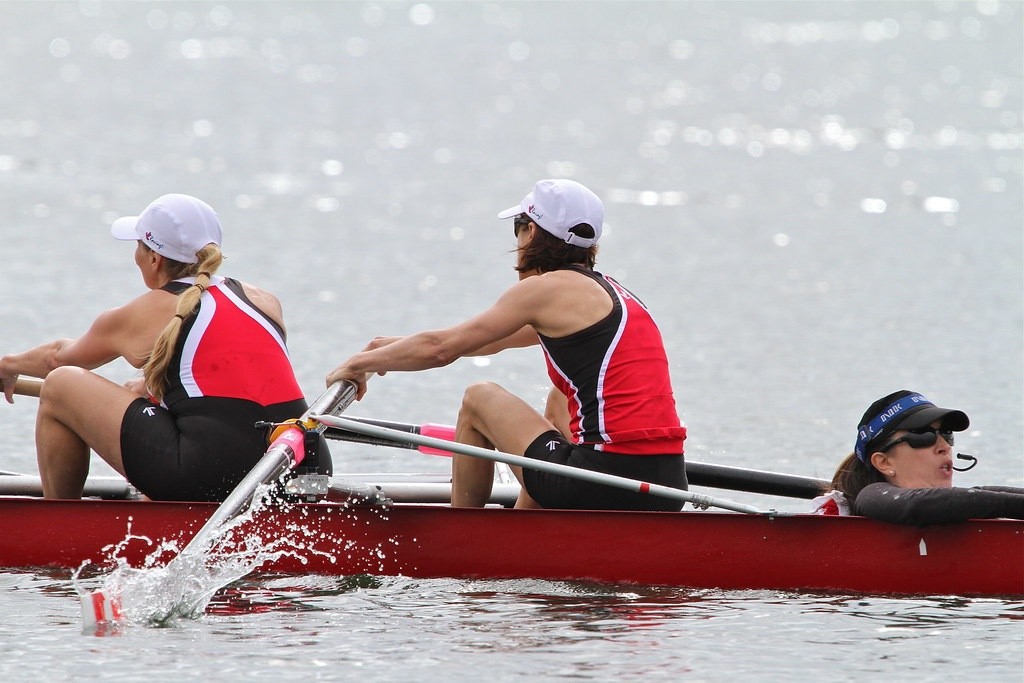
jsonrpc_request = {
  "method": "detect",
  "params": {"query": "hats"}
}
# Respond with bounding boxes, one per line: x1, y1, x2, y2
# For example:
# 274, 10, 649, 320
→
111, 194, 222, 263
865, 404, 970, 453
498, 179, 604, 248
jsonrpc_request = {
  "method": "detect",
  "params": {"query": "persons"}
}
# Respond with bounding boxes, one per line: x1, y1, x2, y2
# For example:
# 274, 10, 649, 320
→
325, 180, 689, 512
0, 193, 332, 502
811, 390, 971, 516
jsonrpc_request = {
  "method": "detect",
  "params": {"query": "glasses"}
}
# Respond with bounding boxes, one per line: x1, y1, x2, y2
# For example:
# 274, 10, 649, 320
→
513, 218, 531, 237
879, 424, 954, 452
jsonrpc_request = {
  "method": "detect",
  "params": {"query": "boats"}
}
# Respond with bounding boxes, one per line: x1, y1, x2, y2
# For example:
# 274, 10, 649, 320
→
0, 496, 1024, 602
